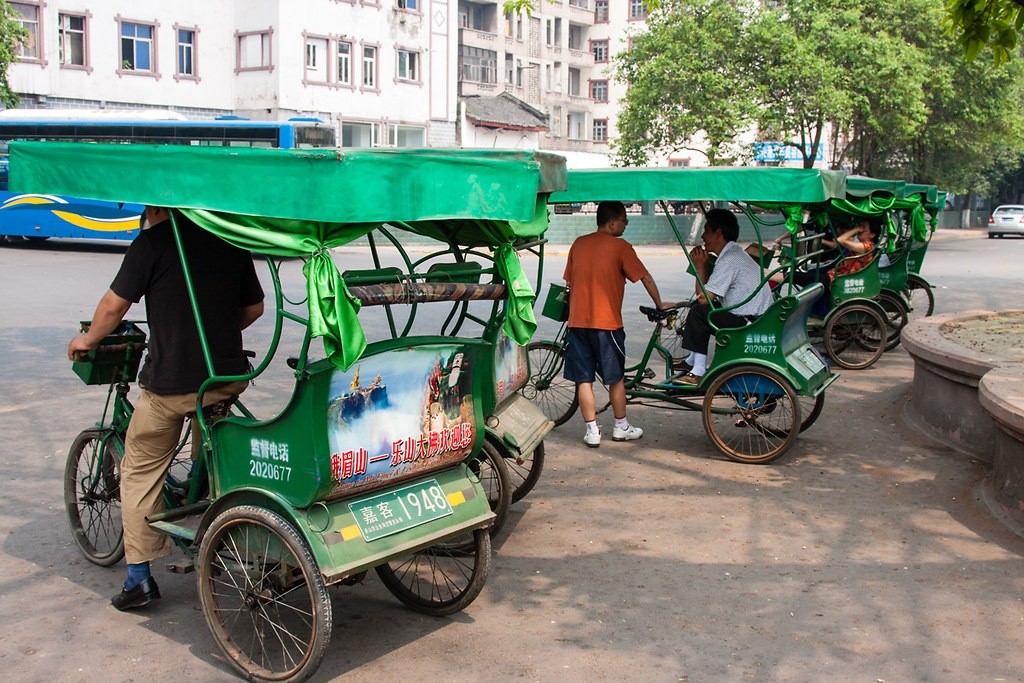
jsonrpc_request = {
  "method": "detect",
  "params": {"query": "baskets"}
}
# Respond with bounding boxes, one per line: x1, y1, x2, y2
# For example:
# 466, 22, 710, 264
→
71, 321, 147, 386
686, 252, 717, 277
541, 283, 570, 322
742, 241, 776, 269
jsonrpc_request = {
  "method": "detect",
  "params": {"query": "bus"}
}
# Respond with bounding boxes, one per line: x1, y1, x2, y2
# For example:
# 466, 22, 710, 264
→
0, 107, 336, 251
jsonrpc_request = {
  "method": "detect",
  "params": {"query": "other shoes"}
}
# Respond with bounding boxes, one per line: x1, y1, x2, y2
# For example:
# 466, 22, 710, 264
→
672, 357, 692, 372
672, 374, 702, 387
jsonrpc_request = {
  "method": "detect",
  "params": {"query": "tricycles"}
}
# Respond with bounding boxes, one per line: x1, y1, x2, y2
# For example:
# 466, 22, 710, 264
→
233, 146, 567, 557
516, 170, 845, 464
758, 176, 947, 368
6, 141, 536, 683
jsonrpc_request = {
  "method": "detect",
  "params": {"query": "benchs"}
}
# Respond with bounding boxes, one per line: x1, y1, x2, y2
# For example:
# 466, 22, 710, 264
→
833, 245, 885, 302
705, 281, 825, 371
212, 267, 502, 507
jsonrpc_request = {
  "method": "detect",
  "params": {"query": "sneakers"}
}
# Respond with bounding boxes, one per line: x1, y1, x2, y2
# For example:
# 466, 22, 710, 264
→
612, 423, 643, 441
583, 428, 601, 448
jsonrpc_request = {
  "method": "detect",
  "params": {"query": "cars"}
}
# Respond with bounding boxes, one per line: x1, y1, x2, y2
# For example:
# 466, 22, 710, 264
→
944, 192, 986, 212
987, 204, 1024, 239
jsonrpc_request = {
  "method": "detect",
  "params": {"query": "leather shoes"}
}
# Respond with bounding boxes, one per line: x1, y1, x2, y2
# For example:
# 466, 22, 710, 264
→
111, 577, 160, 610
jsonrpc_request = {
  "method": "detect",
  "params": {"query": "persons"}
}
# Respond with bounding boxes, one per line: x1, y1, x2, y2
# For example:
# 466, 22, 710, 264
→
672, 208, 775, 386
563, 200, 677, 447
764, 219, 882, 288
68, 204, 266, 610
768, 222, 841, 250
